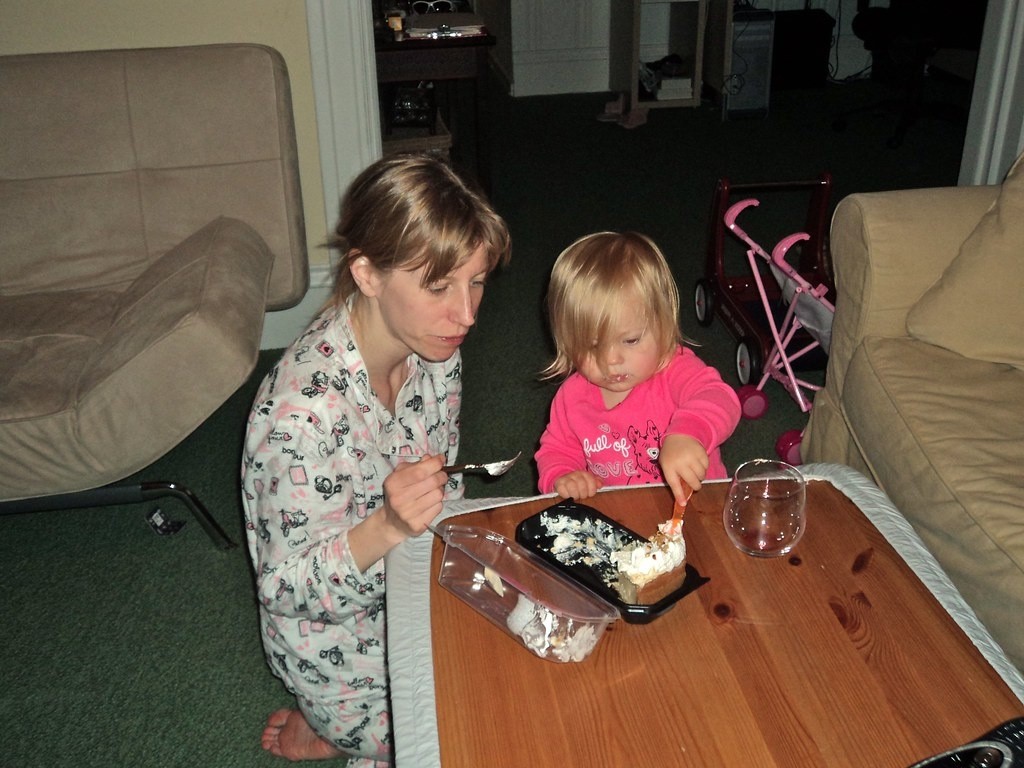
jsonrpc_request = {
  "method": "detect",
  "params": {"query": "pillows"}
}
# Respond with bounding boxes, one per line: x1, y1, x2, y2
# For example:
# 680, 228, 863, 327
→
904, 148, 1023, 375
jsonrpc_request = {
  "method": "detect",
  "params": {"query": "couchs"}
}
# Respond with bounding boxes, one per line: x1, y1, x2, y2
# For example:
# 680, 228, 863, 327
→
795, 184, 1024, 692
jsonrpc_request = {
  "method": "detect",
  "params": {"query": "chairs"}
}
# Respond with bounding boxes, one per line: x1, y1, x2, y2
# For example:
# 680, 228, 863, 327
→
0, 216, 275, 551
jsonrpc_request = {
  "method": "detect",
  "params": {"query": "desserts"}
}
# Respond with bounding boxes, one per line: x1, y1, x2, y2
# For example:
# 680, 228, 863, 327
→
486, 512, 686, 663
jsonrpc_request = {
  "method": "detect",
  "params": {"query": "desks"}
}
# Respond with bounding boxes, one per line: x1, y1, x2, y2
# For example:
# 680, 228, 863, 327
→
375, 27, 498, 211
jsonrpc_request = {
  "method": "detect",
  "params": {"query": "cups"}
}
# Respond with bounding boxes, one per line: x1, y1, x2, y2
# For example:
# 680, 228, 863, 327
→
722, 458, 808, 558
392, 94, 432, 124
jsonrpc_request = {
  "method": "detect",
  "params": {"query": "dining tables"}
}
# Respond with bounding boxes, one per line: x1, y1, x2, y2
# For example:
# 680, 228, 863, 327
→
384, 460, 1023, 768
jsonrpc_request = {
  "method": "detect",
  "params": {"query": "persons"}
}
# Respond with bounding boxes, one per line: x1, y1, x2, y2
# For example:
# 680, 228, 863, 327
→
238, 147, 510, 768
533, 229, 743, 509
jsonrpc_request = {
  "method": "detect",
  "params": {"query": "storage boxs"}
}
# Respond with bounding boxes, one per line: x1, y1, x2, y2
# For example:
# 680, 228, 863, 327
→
438, 524, 621, 664
385, 80, 439, 137
379, 113, 452, 169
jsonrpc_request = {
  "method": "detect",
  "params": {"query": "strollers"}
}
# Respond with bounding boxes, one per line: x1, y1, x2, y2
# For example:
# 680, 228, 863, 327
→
725, 197, 841, 468
